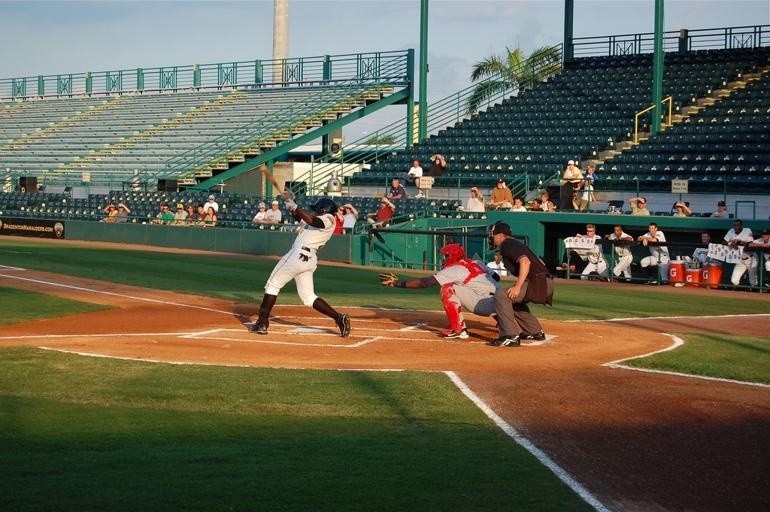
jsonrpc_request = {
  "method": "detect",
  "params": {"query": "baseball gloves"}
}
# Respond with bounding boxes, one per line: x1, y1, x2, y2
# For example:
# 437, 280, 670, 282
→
379, 272, 397, 287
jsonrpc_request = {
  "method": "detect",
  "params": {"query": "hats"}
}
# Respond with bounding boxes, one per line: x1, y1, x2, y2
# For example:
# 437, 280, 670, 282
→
488, 220, 512, 250
176, 204, 183, 208
718, 201, 725, 206
257, 202, 265, 208
272, 201, 279, 205
568, 160, 574, 165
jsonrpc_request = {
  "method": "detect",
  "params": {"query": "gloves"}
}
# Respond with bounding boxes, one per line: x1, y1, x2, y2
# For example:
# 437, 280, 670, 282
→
376, 272, 401, 288
283, 199, 298, 212
281, 187, 296, 202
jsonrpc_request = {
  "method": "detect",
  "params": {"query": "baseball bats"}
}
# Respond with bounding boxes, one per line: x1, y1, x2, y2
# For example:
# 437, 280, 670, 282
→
260, 163, 290, 202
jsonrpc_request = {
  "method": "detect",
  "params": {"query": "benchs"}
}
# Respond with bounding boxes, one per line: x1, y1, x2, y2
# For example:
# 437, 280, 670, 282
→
0, 85, 392, 175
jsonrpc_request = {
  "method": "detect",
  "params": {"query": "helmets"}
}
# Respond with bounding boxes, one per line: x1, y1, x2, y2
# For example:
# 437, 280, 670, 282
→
309, 198, 337, 216
440, 243, 465, 270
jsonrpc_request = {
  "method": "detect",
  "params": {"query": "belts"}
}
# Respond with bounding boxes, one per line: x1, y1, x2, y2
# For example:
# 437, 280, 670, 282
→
302, 246, 310, 252
743, 255, 753, 260
593, 261, 602, 265
489, 292, 494, 295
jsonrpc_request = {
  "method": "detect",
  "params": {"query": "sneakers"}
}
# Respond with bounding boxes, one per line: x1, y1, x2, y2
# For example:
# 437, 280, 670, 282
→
439, 328, 469, 339
519, 329, 545, 341
489, 335, 520, 347
335, 313, 351, 337
249, 324, 268, 334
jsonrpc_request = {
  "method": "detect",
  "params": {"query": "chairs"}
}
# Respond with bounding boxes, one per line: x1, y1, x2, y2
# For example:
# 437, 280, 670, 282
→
344, 48, 769, 234
1, 187, 486, 232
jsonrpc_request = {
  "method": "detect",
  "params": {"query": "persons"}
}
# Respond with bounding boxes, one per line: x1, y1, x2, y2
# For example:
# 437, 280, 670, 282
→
693, 220, 770, 292
249, 187, 351, 337
564, 160, 597, 212
630, 197, 649, 215
673, 201, 692, 216
710, 201, 729, 218
152, 195, 218, 227
561, 223, 670, 288
334, 204, 358, 234
102, 202, 130, 222
367, 156, 446, 225
488, 251, 507, 276
253, 200, 281, 225
377, 242, 505, 338
490, 221, 554, 347
467, 179, 554, 212
